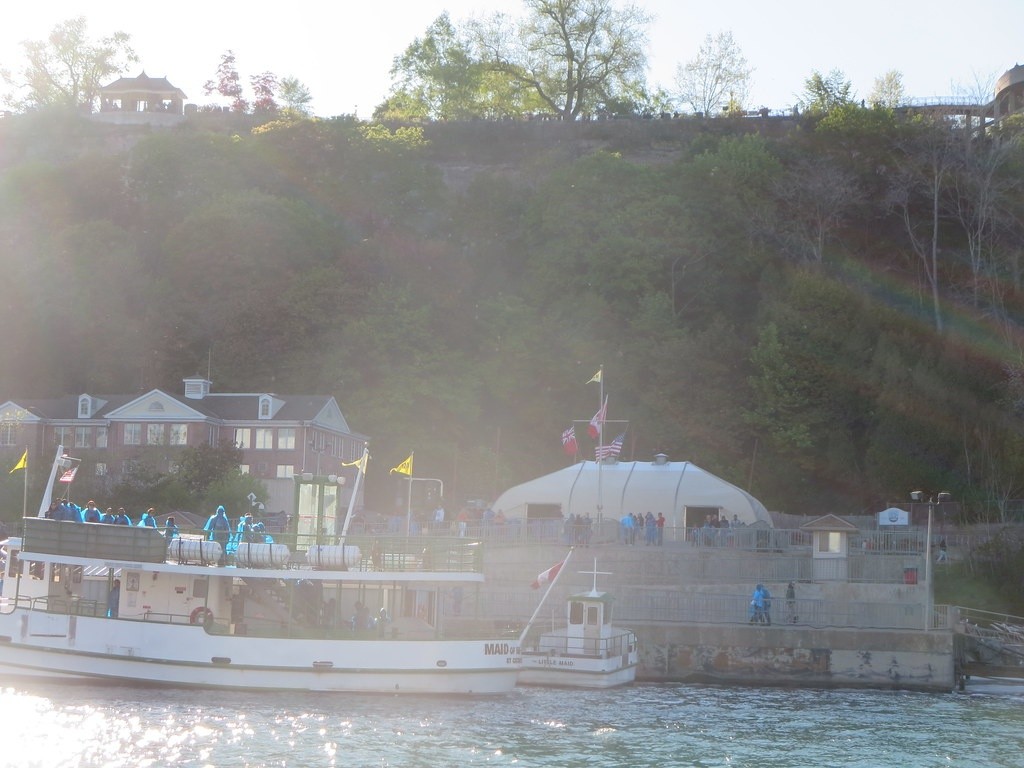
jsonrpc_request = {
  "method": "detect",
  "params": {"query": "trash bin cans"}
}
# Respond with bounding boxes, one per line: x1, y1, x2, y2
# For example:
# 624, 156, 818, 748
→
904, 566, 919, 584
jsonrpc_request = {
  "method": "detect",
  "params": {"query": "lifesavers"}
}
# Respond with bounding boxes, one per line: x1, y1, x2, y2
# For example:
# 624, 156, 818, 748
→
190, 606, 214, 629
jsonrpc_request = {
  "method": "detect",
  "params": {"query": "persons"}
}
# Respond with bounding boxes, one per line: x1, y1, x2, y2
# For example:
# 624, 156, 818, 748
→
937, 536, 947, 562
563, 512, 592, 544
44, 498, 157, 530
621, 511, 665, 547
690, 514, 740, 546
749, 580, 799, 626
163, 516, 178, 545
372, 539, 384, 572
202, 505, 273, 567
325, 597, 391, 631
456, 507, 506, 537
109, 580, 120, 617
392, 505, 444, 535
0, 571, 4, 596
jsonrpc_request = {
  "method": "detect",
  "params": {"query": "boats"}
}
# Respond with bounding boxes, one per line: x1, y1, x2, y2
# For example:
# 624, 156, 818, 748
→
516, 557, 640, 690
0, 527, 525, 695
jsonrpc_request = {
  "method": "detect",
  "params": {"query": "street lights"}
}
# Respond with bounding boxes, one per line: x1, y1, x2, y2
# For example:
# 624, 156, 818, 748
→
910, 490, 952, 627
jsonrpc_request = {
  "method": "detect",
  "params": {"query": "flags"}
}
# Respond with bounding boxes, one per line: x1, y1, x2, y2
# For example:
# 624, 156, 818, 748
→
59, 467, 78, 482
594, 432, 625, 463
586, 395, 608, 440
561, 425, 578, 454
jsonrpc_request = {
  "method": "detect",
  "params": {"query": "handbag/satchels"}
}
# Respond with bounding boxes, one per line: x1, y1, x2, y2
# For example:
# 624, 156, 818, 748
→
751, 600, 757, 606
